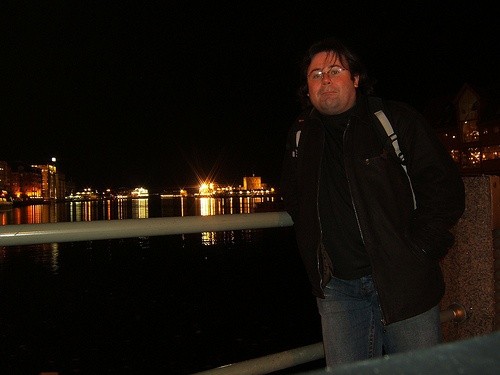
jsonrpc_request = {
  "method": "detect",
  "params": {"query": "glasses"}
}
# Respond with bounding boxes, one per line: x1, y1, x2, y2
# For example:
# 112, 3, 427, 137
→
307, 67, 351, 80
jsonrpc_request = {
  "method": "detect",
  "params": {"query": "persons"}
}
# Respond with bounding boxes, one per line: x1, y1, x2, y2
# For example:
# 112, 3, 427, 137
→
282, 38, 467, 372
